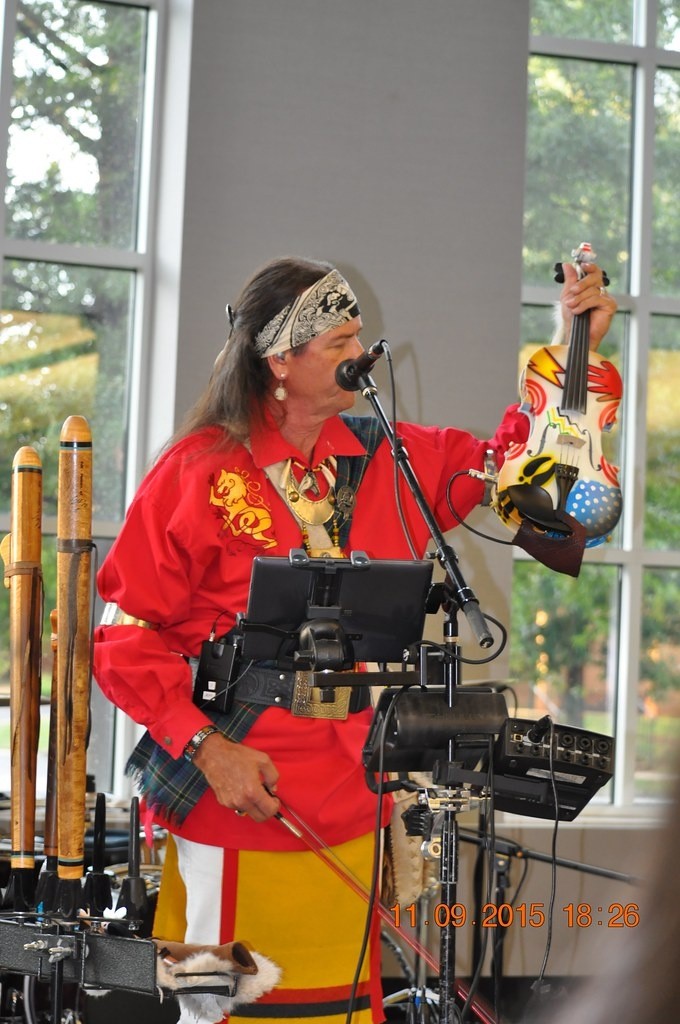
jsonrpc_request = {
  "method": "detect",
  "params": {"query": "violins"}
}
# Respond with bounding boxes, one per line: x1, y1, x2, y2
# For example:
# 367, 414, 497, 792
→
489, 242, 623, 548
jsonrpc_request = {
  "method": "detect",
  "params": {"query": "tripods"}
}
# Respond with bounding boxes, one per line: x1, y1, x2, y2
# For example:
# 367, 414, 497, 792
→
382, 893, 462, 1024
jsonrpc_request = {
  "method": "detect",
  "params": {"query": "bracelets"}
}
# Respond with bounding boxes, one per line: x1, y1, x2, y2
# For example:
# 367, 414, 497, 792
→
182, 725, 220, 762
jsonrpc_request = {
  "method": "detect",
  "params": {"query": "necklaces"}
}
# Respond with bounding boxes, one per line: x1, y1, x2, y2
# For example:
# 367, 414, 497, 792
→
278, 459, 345, 558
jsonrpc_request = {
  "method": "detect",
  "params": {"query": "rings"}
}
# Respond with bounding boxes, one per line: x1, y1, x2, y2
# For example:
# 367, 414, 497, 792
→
235, 809, 245, 816
598, 286, 606, 297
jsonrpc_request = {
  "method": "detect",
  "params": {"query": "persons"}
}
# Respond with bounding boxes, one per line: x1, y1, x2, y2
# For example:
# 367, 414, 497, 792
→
92, 256, 617, 1024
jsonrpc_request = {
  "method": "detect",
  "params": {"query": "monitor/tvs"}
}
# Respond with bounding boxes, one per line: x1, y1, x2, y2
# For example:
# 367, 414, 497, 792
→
242, 555, 434, 665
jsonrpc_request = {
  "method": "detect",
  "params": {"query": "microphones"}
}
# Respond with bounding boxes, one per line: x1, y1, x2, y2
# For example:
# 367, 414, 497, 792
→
334, 340, 389, 393
